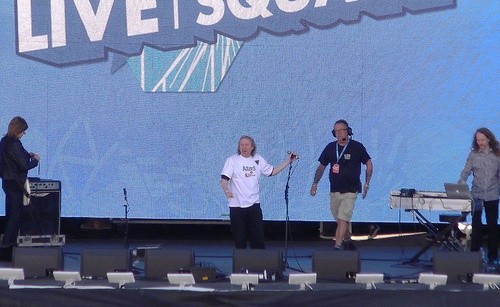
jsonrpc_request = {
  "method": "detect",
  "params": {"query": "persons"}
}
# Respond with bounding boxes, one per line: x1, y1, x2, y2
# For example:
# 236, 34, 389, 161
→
458, 127, 500, 261
0, 116, 40, 244
220, 135, 298, 248
311, 119, 373, 250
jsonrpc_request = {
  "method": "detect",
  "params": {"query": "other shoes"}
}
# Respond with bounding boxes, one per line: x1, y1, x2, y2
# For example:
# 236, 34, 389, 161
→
486, 260, 496, 274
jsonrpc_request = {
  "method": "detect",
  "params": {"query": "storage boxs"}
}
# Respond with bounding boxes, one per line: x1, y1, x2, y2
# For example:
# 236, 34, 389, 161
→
18, 190, 61, 237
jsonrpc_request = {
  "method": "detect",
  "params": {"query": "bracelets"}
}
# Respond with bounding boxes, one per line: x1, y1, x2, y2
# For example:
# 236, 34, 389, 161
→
312, 185, 317, 187
364, 184, 370, 189
225, 191, 229, 194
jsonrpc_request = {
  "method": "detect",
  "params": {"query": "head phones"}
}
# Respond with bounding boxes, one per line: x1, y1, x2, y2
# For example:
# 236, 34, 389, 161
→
332, 120, 352, 137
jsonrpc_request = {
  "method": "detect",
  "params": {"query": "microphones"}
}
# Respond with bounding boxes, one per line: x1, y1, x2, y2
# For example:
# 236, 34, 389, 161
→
123, 188, 127, 199
342, 138, 346, 141
287, 151, 299, 159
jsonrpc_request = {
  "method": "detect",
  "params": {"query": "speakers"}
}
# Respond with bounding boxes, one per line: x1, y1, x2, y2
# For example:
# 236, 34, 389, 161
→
12, 246, 64, 279
18, 179, 62, 235
312, 250, 361, 281
232, 249, 284, 277
81, 249, 132, 279
432, 252, 482, 282
144, 249, 195, 280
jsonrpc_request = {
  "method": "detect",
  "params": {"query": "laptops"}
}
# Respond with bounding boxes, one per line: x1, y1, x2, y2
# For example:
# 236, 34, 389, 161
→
444, 182, 473, 198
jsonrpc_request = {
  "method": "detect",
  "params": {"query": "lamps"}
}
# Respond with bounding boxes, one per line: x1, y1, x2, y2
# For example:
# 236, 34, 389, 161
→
288, 273, 317, 290
0, 268, 25, 286
230, 273, 259, 290
53, 271, 82, 288
106, 272, 136, 289
472, 273, 500, 292
418, 272, 449, 291
354, 273, 384, 289
167, 273, 196, 289
